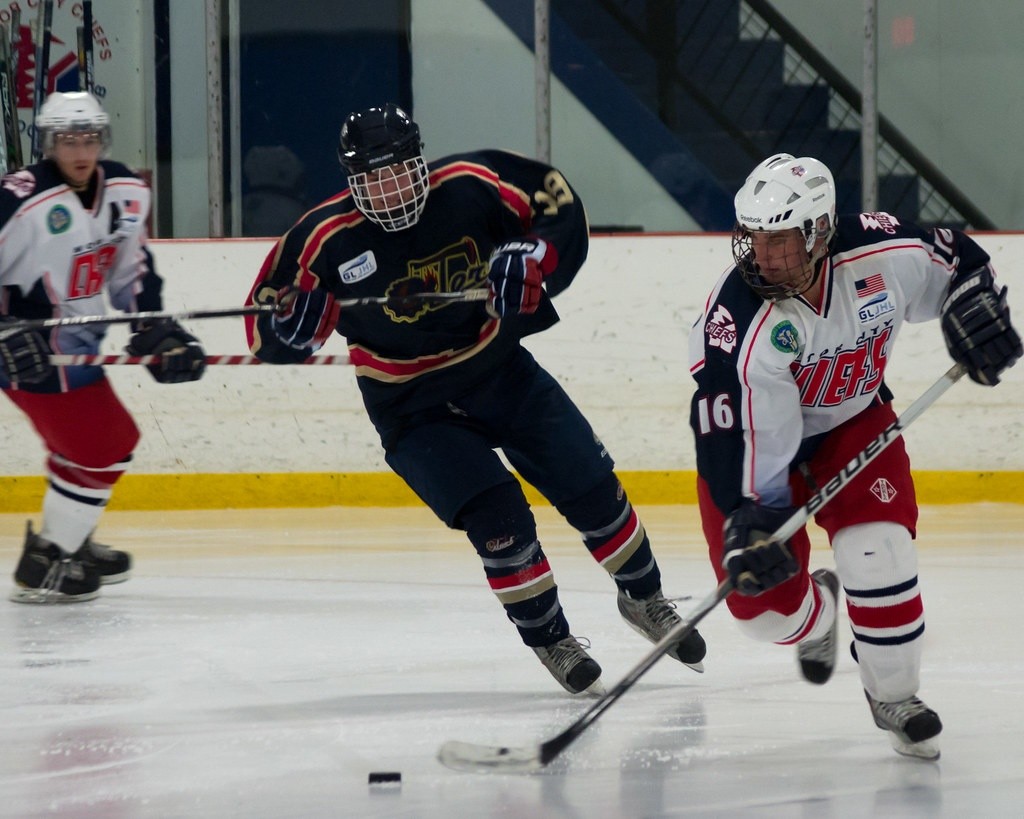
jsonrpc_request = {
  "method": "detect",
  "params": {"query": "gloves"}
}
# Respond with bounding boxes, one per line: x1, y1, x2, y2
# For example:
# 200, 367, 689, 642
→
133, 315, 207, 384
258, 282, 338, 367
486, 233, 556, 316
0, 314, 53, 388
721, 505, 797, 598
933, 284, 1021, 390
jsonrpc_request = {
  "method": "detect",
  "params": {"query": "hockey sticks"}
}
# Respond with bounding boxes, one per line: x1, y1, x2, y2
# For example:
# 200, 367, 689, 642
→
433, 357, 968, 778
44, 349, 357, 370
0, 285, 495, 333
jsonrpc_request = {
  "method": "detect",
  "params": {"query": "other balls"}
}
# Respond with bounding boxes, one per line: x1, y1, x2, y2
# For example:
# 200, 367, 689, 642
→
366, 769, 405, 788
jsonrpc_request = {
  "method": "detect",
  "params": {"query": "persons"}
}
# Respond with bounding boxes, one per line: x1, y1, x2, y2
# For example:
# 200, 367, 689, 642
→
689, 153, 1022, 744
0, 91, 208, 605
243, 106, 707, 694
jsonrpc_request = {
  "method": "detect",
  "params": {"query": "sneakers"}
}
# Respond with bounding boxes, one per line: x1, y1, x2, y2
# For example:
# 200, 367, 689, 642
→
69, 531, 135, 586
11, 522, 105, 606
866, 682, 947, 767
610, 580, 707, 675
532, 620, 610, 708
792, 572, 839, 687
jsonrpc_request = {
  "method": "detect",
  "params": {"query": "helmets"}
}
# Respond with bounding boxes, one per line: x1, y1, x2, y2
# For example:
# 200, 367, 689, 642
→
726, 150, 836, 294
31, 85, 111, 178
334, 103, 435, 232
